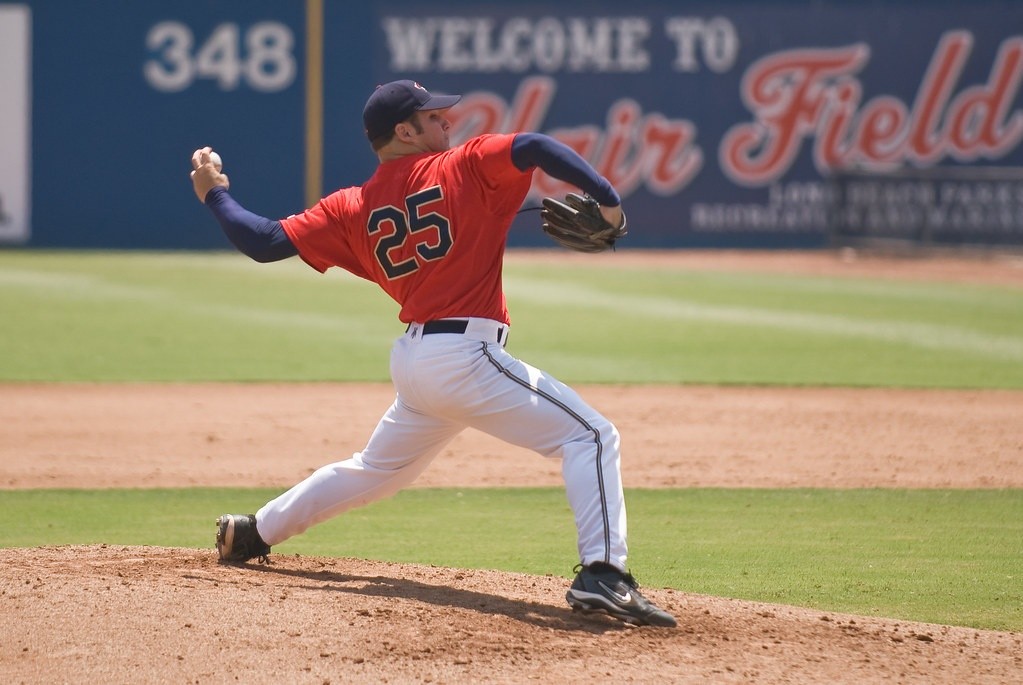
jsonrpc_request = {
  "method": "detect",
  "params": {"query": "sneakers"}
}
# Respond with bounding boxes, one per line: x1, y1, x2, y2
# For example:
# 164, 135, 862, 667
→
214, 514, 270, 565
566, 561, 678, 628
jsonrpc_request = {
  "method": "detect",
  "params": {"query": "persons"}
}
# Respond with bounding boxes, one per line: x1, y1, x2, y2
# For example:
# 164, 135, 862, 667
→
190, 79, 677, 625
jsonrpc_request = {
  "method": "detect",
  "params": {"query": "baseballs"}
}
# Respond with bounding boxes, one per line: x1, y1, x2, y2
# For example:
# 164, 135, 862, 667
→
200, 151, 223, 174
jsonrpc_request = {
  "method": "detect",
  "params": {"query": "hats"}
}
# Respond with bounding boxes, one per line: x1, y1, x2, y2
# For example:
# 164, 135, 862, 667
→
362, 79, 461, 142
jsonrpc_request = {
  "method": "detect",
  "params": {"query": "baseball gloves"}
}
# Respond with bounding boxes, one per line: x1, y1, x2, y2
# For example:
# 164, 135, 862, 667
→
537, 189, 630, 255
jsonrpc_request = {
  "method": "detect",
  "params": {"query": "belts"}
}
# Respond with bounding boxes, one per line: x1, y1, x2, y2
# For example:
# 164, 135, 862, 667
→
406, 321, 509, 347
